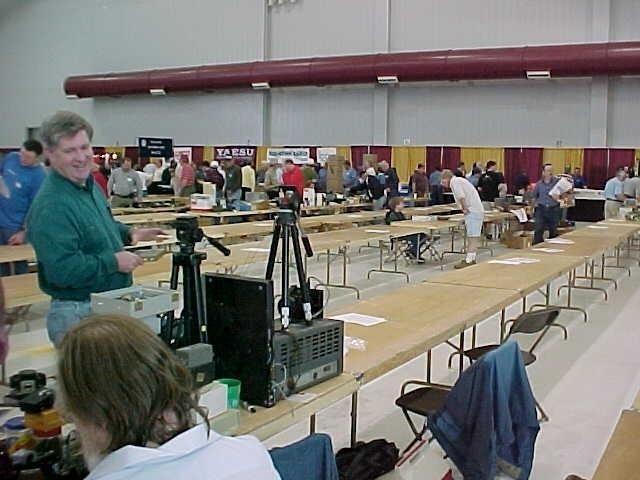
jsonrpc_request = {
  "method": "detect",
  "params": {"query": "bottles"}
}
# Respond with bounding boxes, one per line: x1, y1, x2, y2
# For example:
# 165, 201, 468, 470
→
1, 416, 32, 453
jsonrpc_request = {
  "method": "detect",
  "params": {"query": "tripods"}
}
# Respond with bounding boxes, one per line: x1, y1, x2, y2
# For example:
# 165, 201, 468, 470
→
164, 252, 209, 350
265, 216, 313, 330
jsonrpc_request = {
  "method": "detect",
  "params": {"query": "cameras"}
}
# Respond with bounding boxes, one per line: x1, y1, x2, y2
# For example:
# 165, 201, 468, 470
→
10, 368, 47, 397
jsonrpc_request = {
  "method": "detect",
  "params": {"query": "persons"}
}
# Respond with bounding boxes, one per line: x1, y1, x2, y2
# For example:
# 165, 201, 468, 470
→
1, 281, 22, 479
387, 197, 440, 264
93, 153, 357, 212
440, 169, 485, 269
350, 153, 399, 210
514, 164, 588, 245
25, 110, 171, 345
54, 314, 284, 479
0, 139, 48, 277
604, 165, 639, 223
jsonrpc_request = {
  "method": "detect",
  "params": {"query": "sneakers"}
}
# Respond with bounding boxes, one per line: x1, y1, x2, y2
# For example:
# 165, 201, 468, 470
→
455, 260, 476, 269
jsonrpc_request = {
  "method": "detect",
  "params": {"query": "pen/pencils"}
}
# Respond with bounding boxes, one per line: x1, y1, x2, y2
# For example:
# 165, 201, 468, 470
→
239, 400, 257, 412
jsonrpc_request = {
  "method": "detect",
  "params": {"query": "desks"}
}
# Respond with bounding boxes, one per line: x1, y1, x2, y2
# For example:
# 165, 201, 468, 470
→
0, 193, 640, 480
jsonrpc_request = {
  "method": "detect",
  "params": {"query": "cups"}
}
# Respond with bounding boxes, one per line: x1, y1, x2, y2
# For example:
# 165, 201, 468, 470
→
217, 378, 241, 408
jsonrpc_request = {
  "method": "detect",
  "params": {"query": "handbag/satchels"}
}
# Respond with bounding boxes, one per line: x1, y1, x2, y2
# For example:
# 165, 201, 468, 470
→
335, 439, 402, 480
